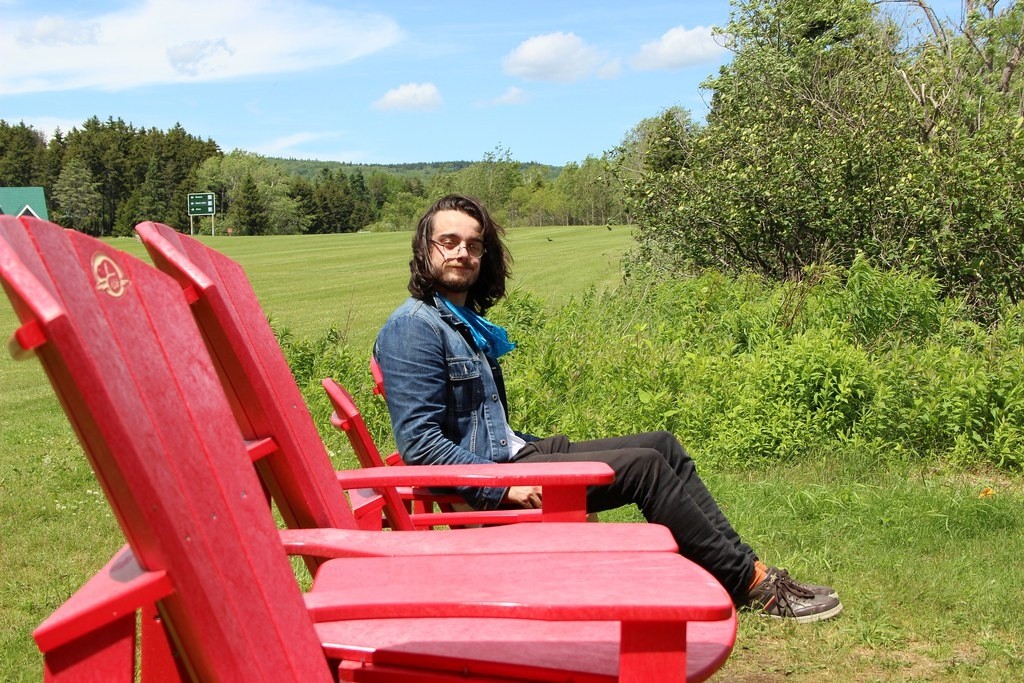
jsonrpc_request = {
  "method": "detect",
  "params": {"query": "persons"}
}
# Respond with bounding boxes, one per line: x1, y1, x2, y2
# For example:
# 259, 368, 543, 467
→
373, 193, 843, 623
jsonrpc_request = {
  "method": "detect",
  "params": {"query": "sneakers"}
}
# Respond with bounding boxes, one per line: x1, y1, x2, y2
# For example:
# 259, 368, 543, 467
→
765, 566, 839, 598
739, 568, 843, 622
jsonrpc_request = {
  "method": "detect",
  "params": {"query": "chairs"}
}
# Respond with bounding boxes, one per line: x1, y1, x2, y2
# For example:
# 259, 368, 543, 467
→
0, 210, 738, 683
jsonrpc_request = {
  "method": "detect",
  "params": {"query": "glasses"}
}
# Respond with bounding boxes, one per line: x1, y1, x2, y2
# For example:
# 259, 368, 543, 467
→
428, 239, 487, 259
